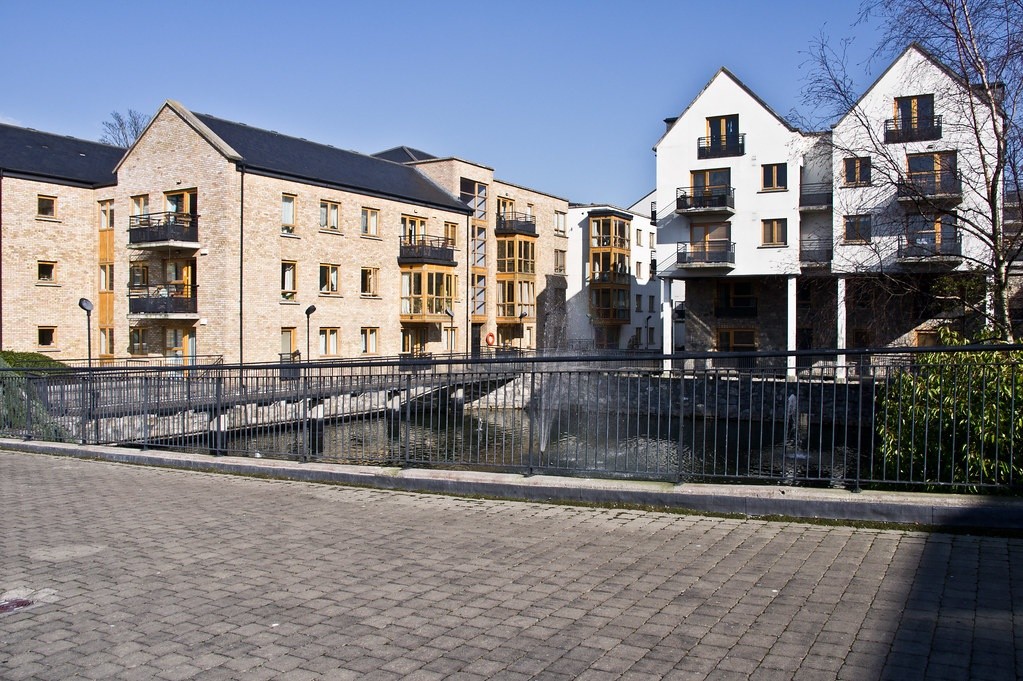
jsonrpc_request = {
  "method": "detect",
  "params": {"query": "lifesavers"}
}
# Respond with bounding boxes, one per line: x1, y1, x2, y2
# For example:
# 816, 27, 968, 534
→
486, 333, 495, 346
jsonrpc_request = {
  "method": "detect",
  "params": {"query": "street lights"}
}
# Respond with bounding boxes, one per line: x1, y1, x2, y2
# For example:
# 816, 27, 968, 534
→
306, 304, 315, 389
79, 298, 94, 410
445, 308, 453, 375
587, 313, 594, 350
518, 311, 527, 361
646, 315, 652, 348
